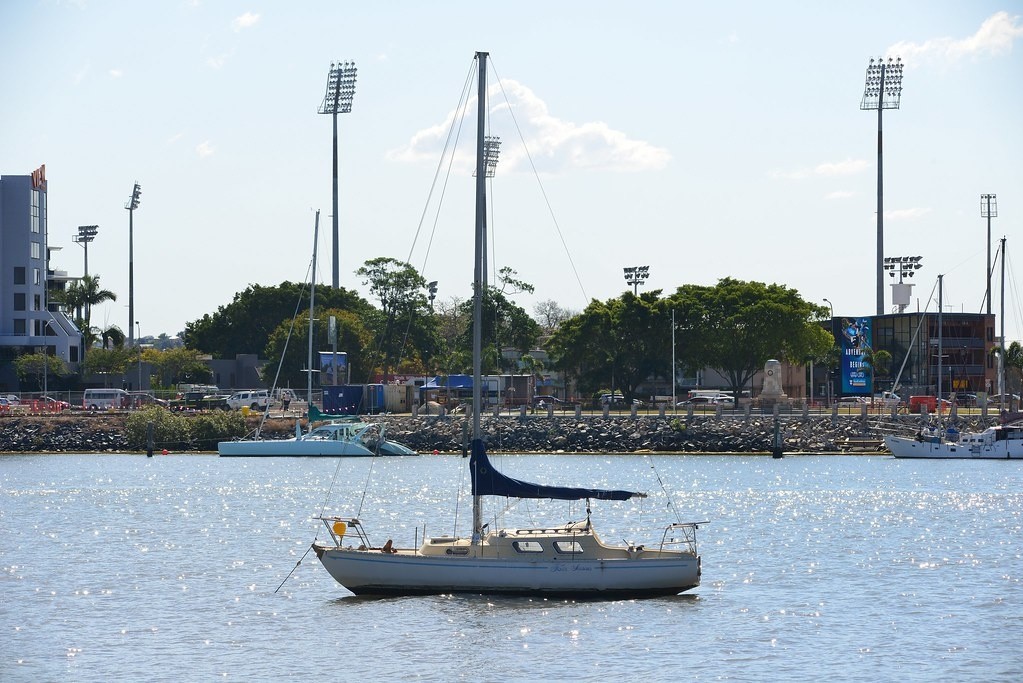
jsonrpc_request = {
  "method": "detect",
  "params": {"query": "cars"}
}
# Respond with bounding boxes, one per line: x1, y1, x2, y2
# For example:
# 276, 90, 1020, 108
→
0, 394, 19, 417
123, 393, 167, 409
676, 396, 717, 411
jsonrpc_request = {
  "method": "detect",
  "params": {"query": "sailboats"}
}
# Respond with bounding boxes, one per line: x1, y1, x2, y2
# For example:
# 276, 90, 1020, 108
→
218, 213, 421, 458
309, 49, 706, 600
882, 235, 1023, 461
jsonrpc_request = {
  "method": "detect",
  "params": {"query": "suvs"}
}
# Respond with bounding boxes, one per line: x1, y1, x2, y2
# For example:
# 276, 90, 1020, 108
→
533, 395, 571, 410
601, 396, 643, 410
221, 390, 276, 413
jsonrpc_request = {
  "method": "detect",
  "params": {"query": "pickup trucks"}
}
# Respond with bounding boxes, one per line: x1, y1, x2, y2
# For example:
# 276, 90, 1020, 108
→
19, 396, 72, 410
169, 392, 228, 413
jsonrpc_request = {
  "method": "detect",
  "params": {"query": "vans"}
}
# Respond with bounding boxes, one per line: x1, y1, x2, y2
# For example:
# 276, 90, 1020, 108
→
83, 388, 130, 411
599, 393, 626, 409
712, 396, 737, 410
649, 395, 677, 409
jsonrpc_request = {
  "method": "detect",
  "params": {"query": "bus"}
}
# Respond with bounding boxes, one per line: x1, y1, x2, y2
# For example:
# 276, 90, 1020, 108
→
688, 389, 752, 405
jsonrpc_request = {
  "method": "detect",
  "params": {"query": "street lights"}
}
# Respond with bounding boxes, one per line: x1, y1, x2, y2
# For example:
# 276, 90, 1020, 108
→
470, 137, 503, 302
979, 193, 999, 316
44, 319, 54, 397
123, 182, 143, 344
823, 298, 834, 336
70, 225, 99, 336
315, 61, 358, 299
859, 56, 904, 316
884, 256, 922, 314
623, 265, 650, 299
